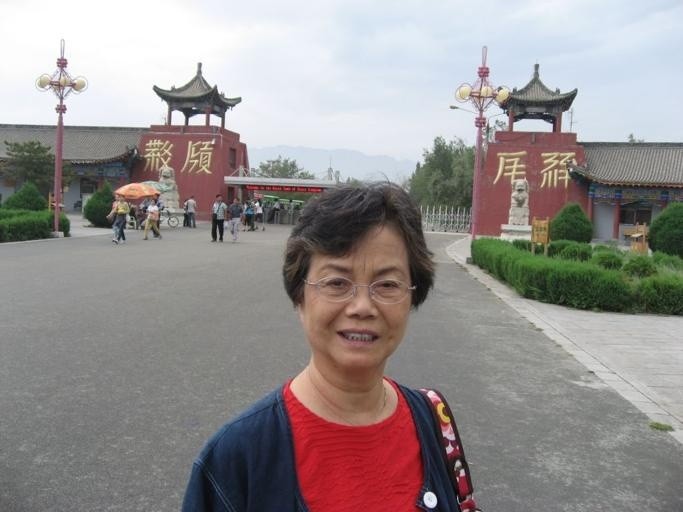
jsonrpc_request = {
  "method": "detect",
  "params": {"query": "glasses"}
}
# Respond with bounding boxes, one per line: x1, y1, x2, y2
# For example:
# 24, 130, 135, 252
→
302, 276, 416, 304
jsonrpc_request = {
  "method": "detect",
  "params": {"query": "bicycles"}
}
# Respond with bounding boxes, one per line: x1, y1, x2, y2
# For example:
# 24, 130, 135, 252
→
159, 206, 179, 228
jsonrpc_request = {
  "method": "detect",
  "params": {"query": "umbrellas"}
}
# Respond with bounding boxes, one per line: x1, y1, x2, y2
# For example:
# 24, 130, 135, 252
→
113, 179, 175, 207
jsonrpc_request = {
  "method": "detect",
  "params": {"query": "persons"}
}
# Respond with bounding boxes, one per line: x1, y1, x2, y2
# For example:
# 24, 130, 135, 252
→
183, 191, 301, 242
110, 190, 164, 244
181, 178, 484, 512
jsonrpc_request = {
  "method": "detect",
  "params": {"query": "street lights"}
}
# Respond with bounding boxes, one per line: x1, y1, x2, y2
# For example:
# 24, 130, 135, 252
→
31, 36, 89, 238
453, 44, 513, 239
446, 104, 507, 145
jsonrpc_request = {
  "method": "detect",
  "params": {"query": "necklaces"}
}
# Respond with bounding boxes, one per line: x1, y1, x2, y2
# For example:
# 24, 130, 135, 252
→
304, 363, 387, 426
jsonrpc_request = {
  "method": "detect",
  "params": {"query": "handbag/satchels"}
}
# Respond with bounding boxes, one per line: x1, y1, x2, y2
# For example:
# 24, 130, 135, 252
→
213, 214, 217, 220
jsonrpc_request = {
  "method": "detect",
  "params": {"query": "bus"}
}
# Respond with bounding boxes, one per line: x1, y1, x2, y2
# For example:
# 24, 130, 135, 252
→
260, 194, 280, 205
277, 198, 305, 209
